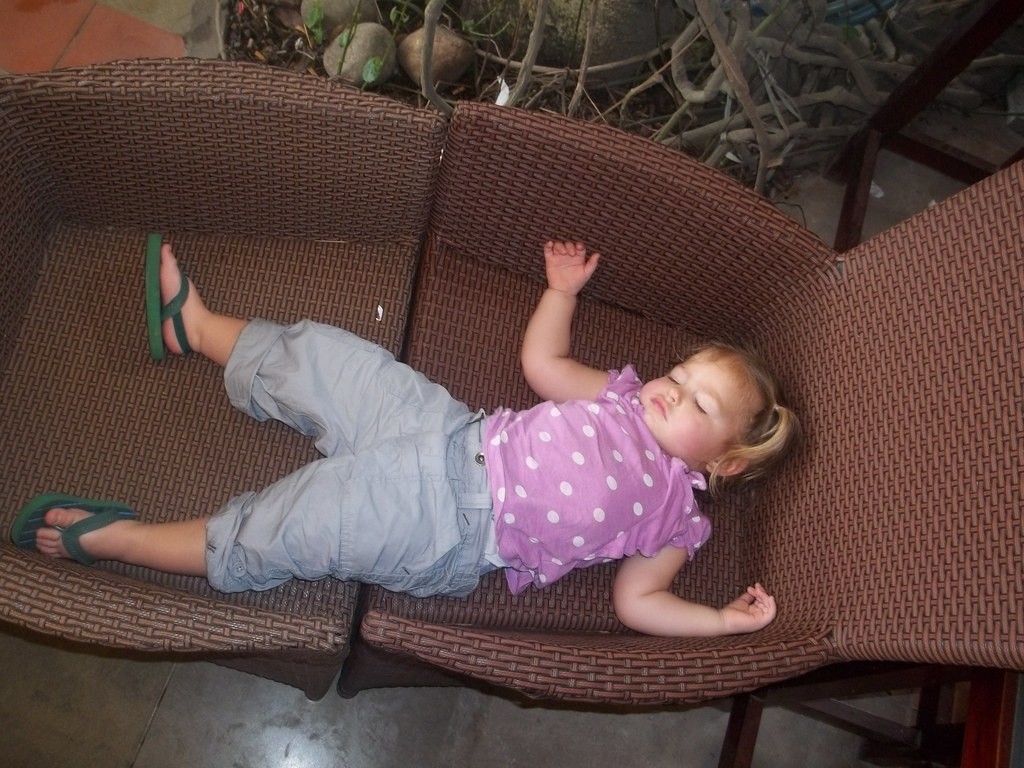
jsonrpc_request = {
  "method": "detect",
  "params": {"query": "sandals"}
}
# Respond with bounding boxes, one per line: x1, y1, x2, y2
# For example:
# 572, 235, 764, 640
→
10, 493, 137, 566
145, 233, 192, 360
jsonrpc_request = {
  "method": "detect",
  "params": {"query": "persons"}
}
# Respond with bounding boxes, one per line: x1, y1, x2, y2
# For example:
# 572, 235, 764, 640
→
9, 235, 797, 637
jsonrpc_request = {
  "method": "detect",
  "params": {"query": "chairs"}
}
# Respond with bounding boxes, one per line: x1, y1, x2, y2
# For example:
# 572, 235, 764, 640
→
0, 58, 448, 701
337, 102, 1024, 768
